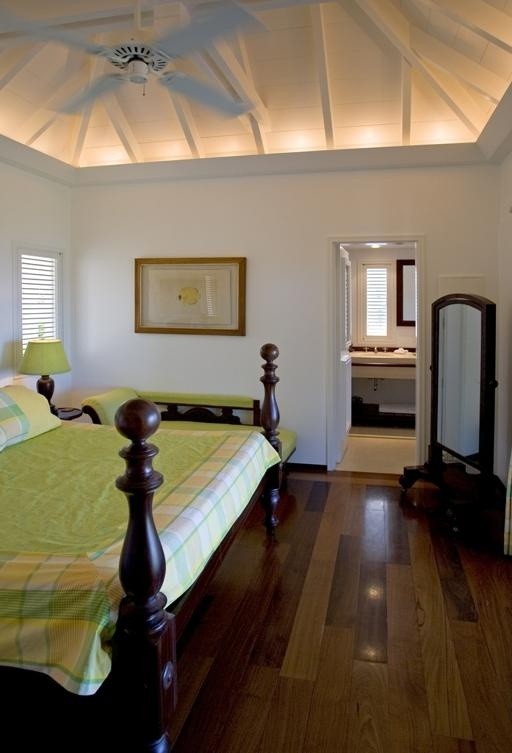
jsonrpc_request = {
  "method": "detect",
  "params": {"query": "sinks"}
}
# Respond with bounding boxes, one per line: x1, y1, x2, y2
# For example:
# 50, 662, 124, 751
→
359, 353, 394, 356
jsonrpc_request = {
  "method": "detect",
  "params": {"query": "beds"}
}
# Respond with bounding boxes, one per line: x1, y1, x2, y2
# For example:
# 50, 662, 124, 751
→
0, 345, 281, 664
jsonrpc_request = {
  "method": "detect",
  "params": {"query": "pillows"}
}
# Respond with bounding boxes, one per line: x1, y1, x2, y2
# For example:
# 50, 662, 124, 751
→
0, 385, 61, 452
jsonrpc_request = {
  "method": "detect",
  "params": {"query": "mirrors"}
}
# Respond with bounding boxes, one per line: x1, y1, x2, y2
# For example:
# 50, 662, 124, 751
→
429, 291, 497, 470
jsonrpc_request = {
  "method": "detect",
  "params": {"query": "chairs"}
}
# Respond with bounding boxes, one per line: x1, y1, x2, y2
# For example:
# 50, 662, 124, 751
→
80, 385, 296, 469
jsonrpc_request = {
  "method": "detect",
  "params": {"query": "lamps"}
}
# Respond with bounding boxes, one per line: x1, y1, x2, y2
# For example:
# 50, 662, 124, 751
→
19, 339, 70, 417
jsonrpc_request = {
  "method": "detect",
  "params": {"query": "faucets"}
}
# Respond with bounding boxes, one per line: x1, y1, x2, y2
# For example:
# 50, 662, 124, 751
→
374, 346, 378, 353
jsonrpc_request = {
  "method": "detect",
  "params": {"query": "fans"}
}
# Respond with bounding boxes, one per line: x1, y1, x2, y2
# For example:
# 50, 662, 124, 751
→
0, 1, 270, 120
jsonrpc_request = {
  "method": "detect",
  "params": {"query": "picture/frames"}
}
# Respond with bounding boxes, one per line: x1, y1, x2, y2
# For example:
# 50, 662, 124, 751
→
133, 257, 245, 337
395, 258, 416, 325
